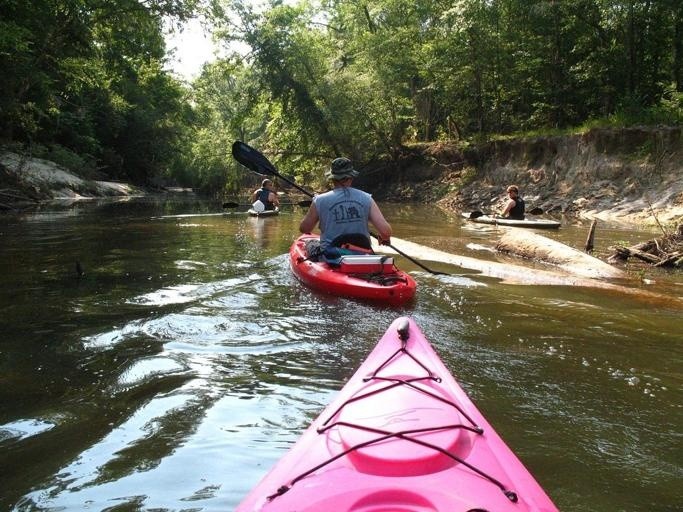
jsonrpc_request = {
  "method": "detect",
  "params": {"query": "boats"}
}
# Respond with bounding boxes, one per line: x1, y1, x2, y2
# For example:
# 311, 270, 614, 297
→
286, 229, 418, 308
247, 206, 280, 217
458, 208, 562, 230
227, 310, 565, 512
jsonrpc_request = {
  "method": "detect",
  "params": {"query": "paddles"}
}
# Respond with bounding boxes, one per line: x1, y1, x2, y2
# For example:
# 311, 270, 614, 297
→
232, 141, 451, 276
223, 202, 312, 208
471, 208, 544, 217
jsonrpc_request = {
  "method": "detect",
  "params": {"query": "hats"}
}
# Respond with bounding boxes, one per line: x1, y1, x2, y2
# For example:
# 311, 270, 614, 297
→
323, 156, 359, 180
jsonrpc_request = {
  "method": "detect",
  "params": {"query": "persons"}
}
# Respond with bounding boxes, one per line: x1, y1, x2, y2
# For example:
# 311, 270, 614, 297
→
251, 178, 280, 210
298, 157, 394, 259
495, 184, 526, 220
